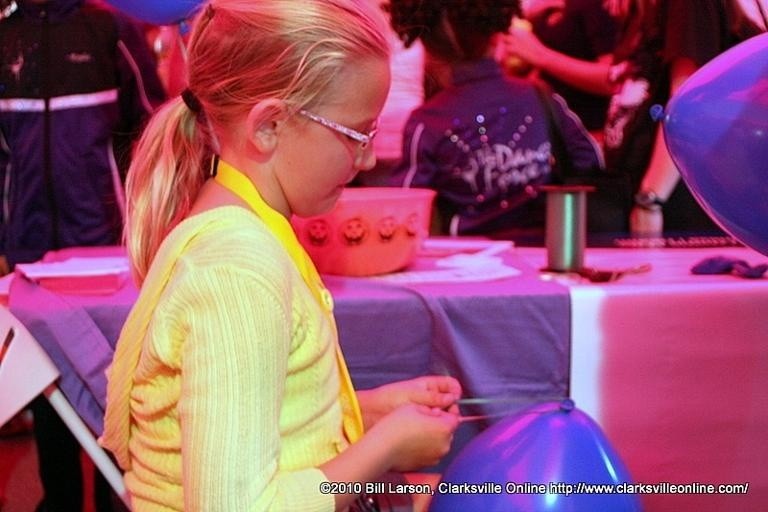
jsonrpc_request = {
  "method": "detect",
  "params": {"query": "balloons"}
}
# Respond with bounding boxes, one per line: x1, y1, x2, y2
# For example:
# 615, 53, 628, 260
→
106, 0, 206, 35
428, 399, 643, 512
650, 30, 768, 257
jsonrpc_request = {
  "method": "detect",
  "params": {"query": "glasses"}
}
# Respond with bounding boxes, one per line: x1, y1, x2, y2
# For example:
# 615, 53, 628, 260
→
299, 109, 380, 150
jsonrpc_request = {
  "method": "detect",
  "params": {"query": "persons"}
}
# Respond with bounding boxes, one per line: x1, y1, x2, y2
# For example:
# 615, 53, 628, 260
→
350, 0, 768, 233
96, 0, 462, 512
0, 0, 171, 512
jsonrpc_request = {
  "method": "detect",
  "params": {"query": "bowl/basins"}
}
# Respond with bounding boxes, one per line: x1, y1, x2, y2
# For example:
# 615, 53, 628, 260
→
283, 187, 437, 278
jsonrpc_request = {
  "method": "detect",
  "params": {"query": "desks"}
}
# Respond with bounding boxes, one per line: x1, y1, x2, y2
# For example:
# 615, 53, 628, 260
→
0, 233, 763, 509
